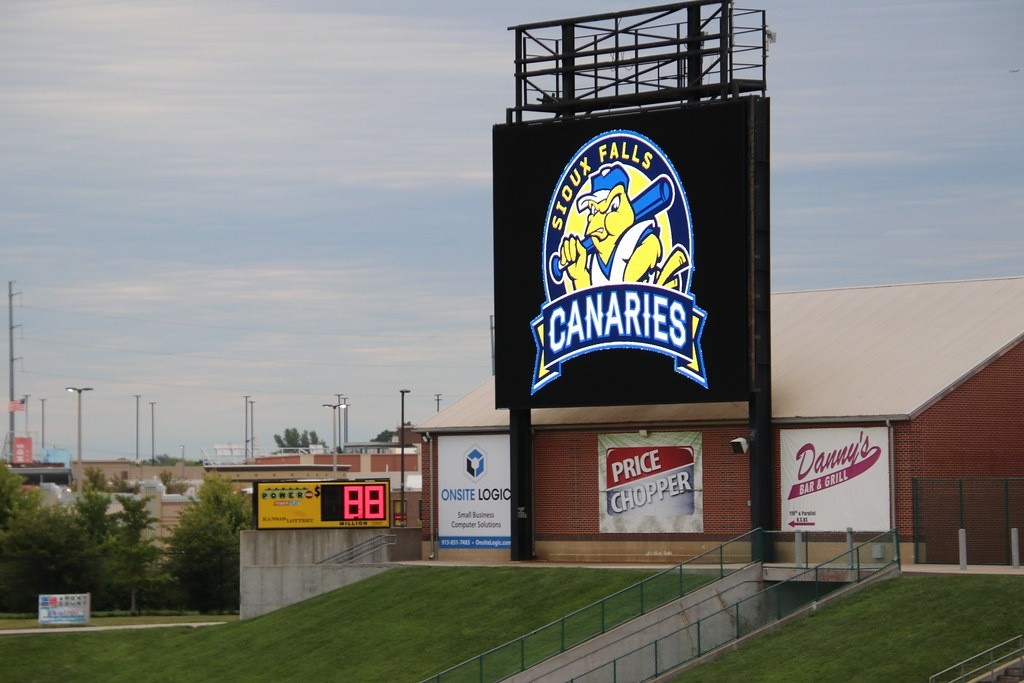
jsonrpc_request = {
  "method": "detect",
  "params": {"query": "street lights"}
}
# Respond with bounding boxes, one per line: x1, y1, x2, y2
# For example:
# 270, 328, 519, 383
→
66, 387, 95, 493
400, 389, 411, 526
149, 402, 156, 465
323, 403, 346, 473
134, 394, 141, 463
39, 398, 47, 450
334, 393, 351, 454
242, 395, 254, 464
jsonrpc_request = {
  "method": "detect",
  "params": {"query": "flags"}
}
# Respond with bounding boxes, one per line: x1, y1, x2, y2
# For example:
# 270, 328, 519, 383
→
8, 398, 26, 412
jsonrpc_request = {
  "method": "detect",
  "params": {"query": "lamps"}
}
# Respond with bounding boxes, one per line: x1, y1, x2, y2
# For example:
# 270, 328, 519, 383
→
729, 436, 750, 455
